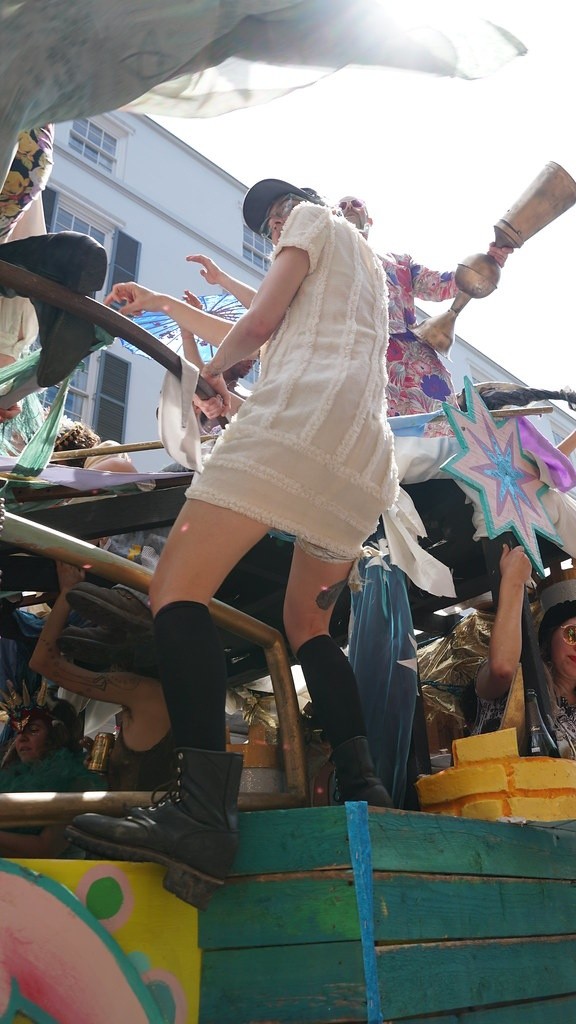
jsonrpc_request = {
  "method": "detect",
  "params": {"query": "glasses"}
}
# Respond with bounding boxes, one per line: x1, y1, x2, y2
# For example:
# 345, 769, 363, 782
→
258, 193, 307, 238
339, 199, 363, 210
560, 624, 576, 646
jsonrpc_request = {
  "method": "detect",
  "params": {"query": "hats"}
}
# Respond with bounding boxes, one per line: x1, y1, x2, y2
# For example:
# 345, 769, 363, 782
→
243, 179, 328, 240
538, 600, 576, 662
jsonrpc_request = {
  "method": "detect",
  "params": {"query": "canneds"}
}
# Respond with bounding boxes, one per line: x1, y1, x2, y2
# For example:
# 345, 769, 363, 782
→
88, 731, 115, 773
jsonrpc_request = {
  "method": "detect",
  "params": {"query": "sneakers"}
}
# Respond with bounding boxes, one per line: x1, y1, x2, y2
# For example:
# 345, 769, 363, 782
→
65, 582, 152, 634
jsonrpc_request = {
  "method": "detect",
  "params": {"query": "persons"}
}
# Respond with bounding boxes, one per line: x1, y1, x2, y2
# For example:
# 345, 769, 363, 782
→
63, 178, 393, 912
452, 542, 576, 760
0, 676, 101, 791
0, 122, 492, 774
334, 193, 514, 415
28, 555, 180, 791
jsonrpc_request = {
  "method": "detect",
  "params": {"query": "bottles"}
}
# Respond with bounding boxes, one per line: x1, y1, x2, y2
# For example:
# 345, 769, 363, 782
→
519, 688, 560, 756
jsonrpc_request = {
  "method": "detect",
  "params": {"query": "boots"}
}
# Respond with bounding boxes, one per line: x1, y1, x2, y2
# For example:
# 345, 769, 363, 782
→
328, 736, 394, 808
63, 747, 244, 908
0, 231, 108, 387
